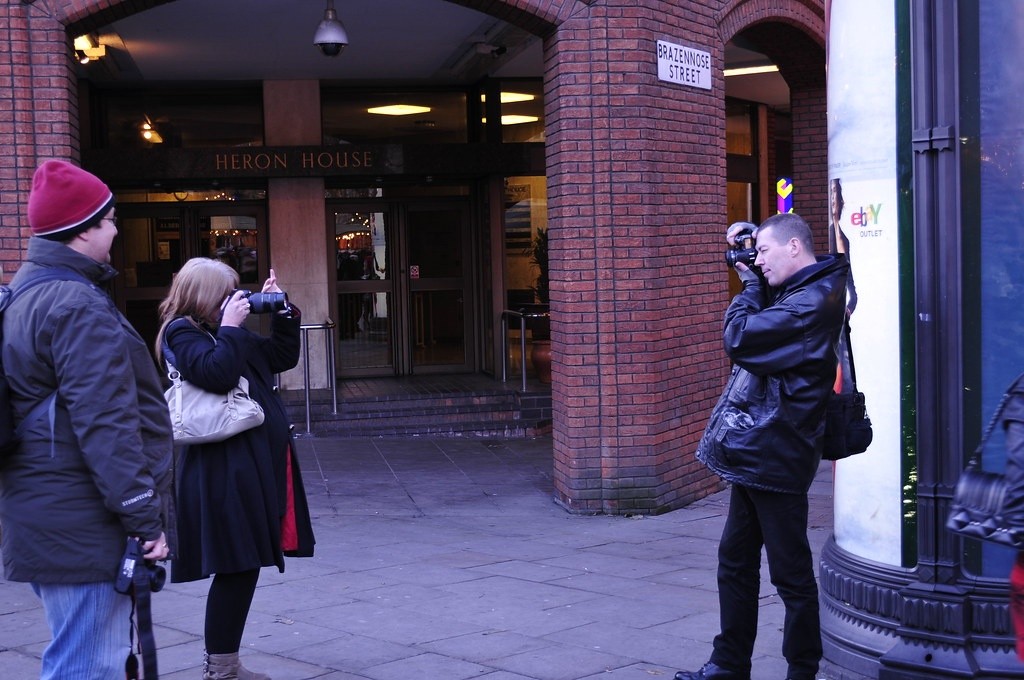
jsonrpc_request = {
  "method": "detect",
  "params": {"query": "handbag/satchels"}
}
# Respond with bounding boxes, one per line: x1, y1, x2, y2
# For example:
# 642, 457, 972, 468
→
821, 392, 872, 460
945, 373, 1023, 550
164, 317, 265, 445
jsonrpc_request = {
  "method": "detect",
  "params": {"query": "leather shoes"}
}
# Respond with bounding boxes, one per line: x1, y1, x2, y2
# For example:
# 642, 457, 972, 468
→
674, 660, 749, 680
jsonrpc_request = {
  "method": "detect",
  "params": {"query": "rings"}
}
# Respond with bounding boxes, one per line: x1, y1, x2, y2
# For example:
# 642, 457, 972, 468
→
163, 543, 167, 547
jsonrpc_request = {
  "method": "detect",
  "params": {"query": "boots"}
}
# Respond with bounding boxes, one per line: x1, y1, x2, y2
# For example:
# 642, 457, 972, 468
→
200, 649, 271, 680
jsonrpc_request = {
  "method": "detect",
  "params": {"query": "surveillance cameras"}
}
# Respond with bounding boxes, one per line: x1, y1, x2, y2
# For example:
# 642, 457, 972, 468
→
312, 18, 350, 57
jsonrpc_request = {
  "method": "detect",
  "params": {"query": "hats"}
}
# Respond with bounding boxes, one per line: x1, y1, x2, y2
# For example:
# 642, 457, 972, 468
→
27, 160, 115, 241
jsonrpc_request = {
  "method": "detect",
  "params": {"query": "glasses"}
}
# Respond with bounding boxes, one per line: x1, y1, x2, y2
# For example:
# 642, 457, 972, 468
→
102, 216, 118, 226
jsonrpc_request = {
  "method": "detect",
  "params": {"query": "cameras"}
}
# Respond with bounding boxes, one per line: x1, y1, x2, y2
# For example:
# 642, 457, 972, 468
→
227, 288, 289, 314
114, 538, 166, 596
725, 233, 761, 271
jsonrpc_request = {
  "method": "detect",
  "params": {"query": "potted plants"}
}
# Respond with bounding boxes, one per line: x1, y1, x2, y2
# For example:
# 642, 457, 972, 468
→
528, 228, 552, 384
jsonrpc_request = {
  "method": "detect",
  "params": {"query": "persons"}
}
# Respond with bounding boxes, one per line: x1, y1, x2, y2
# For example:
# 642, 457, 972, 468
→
1002, 375, 1024, 664
156, 257, 315, 680
0, 159, 173, 680
830, 178, 857, 395
673, 214, 845, 680
